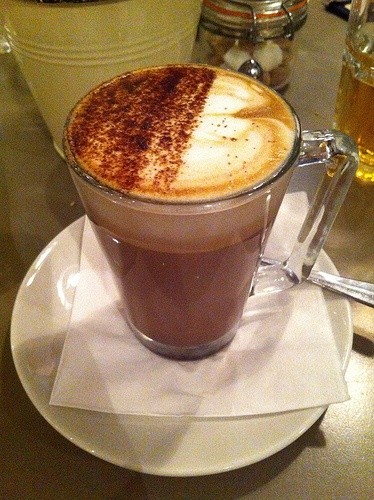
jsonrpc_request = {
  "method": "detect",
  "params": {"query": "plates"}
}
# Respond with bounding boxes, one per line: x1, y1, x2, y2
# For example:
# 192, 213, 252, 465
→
11, 213, 353, 478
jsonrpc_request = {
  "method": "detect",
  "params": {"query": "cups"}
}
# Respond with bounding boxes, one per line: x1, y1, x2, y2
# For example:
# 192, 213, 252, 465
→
325, 0, 374, 185
63, 65, 359, 361
